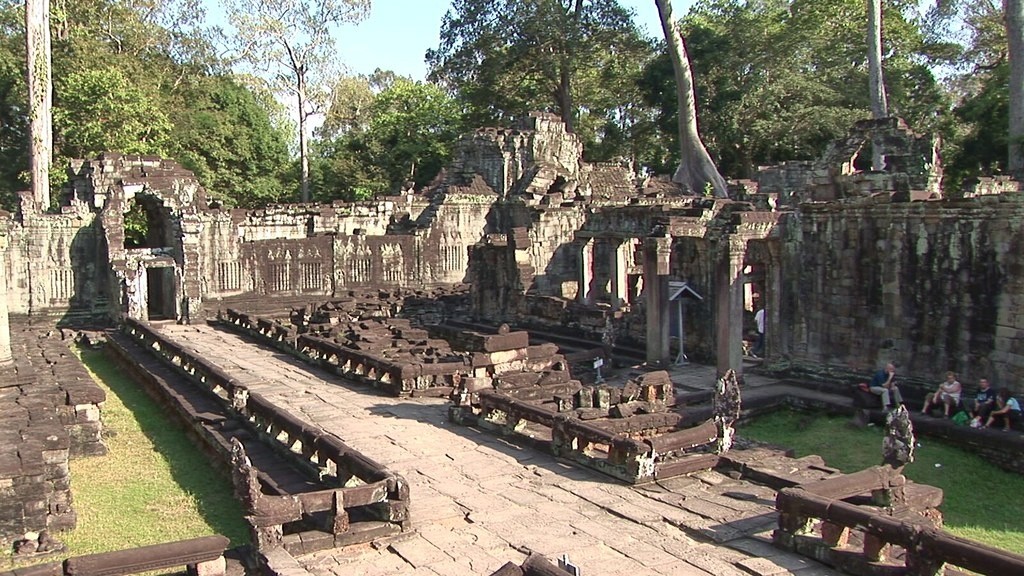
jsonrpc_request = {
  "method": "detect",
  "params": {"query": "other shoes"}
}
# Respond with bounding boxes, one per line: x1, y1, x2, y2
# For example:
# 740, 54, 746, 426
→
1003, 427, 1010, 432
978, 425, 989, 430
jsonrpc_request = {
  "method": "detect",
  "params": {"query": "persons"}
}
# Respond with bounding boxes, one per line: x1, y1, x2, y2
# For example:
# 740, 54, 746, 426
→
750, 303, 765, 359
917, 371, 962, 419
960, 377, 997, 428
869, 363, 903, 414
978, 387, 1022, 432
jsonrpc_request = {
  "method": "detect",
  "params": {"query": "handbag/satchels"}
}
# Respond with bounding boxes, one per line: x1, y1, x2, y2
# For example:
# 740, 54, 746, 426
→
952, 411, 969, 426
